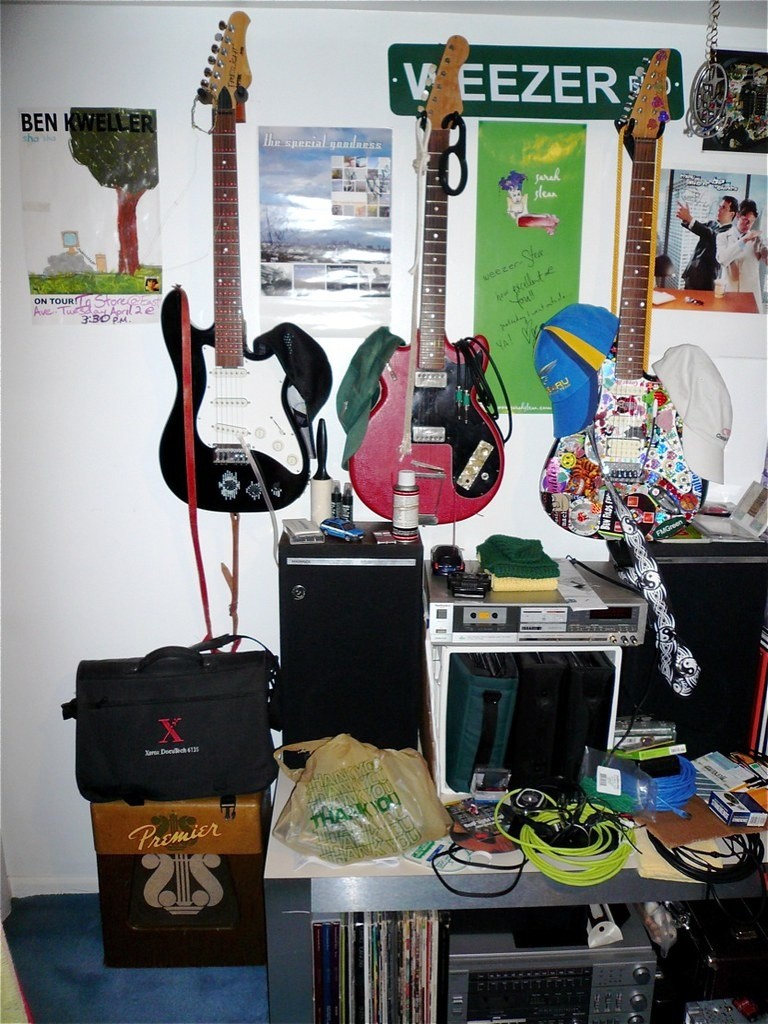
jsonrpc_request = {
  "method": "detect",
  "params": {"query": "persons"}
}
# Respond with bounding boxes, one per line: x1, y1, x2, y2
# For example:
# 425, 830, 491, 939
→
714, 198, 768, 315
675, 195, 739, 290
145, 279, 159, 292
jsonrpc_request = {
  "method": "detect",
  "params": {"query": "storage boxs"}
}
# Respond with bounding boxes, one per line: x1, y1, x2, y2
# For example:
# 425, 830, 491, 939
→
607, 742, 687, 762
90, 786, 272, 968
709, 790, 768, 828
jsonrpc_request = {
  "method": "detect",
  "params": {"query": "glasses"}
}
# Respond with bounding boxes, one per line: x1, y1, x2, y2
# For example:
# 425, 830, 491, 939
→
718, 205, 730, 210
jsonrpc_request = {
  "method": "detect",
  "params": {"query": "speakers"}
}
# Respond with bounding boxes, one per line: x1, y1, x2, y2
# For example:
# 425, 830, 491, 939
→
278, 533, 424, 751
604, 512, 768, 762
91, 791, 267, 968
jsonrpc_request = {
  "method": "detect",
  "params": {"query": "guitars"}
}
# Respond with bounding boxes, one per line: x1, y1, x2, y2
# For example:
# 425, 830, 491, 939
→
349, 35, 505, 526
159, 10, 333, 513
538, 49, 709, 540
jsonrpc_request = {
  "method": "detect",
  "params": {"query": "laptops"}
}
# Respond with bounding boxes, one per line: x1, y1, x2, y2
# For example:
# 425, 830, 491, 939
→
690, 481, 768, 538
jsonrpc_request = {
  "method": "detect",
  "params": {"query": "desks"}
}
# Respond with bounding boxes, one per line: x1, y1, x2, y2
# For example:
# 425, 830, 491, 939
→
654, 288, 760, 313
262, 766, 768, 1024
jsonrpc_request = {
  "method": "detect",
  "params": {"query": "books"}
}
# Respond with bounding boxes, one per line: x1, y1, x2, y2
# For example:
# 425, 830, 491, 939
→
311, 910, 441, 1024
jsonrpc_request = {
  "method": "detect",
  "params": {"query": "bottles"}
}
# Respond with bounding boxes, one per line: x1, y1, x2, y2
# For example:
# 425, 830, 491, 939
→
342, 483, 353, 522
331, 481, 341, 519
393, 470, 419, 542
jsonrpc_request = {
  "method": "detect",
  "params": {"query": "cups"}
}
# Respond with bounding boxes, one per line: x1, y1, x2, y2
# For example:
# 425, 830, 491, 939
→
715, 279, 727, 298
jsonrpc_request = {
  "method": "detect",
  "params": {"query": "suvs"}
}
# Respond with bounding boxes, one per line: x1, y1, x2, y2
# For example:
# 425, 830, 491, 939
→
320, 518, 366, 543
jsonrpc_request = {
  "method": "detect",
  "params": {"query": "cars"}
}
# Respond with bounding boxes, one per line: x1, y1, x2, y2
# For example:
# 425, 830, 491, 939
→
431, 544, 465, 577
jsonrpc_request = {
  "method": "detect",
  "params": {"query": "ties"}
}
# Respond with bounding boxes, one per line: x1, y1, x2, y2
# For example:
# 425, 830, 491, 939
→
712, 225, 720, 230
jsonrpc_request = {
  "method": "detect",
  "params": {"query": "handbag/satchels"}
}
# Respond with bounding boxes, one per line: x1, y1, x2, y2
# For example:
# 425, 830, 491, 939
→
61, 646, 281, 806
271, 733, 455, 865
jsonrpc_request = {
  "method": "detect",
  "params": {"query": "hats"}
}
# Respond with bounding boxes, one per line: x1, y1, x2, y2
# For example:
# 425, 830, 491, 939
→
533, 303, 620, 438
651, 343, 733, 485
253, 321, 334, 458
337, 326, 407, 472
